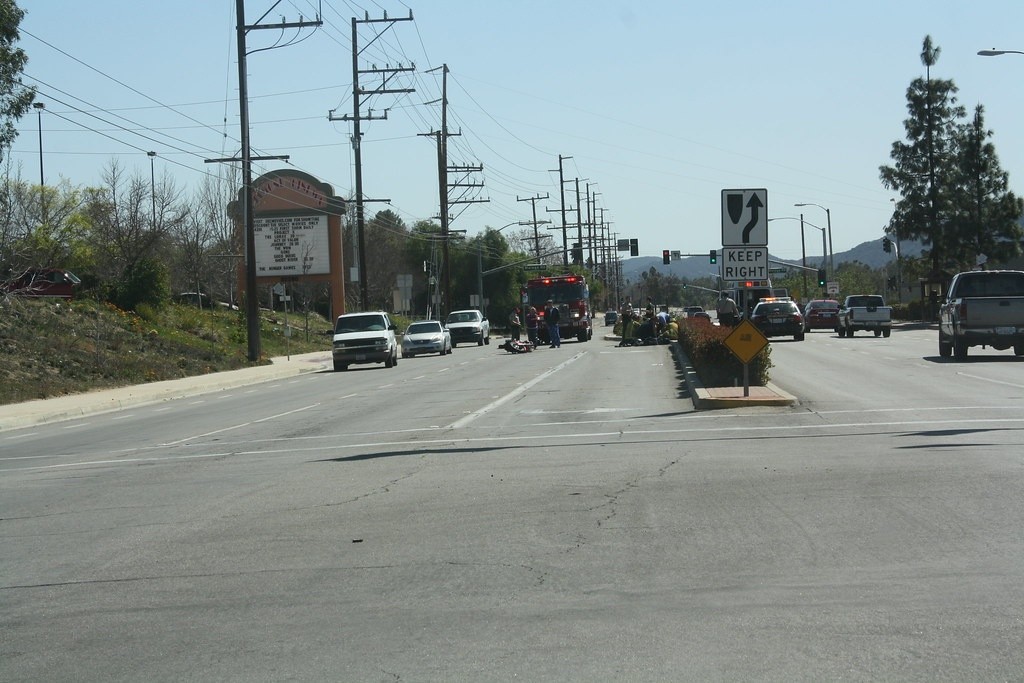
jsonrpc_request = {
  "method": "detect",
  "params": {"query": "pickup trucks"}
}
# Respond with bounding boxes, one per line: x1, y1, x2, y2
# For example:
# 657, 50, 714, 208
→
445, 310, 489, 348
931, 271, 1024, 358
836, 295, 892, 338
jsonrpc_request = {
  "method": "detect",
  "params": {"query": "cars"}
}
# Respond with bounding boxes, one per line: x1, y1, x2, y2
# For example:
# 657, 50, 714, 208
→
750, 297, 806, 341
401, 320, 452, 358
801, 299, 840, 333
174, 293, 237, 310
683, 306, 712, 323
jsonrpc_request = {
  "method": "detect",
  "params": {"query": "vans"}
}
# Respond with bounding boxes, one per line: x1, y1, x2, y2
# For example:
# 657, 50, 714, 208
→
605, 311, 618, 326
326, 312, 398, 371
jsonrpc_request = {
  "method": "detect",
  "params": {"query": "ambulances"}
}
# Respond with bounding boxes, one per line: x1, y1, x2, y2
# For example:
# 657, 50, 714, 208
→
718, 278, 788, 317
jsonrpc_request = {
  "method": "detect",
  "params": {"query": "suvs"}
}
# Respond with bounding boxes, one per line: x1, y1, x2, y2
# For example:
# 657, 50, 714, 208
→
2, 269, 83, 299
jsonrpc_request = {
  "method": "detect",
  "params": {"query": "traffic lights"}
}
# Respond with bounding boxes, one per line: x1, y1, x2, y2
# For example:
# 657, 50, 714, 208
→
663, 250, 670, 265
709, 250, 716, 264
683, 282, 686, 289
887, 277, 892, 289
883, 239, 890, 253
817, 269, 827, 287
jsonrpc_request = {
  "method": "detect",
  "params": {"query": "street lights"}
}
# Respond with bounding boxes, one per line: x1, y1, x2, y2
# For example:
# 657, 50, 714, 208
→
698, 271, 721, 299
768, 217, 828, 298
794, 203, 835, 299
33, 102, 46, 226
890, 198, 901, 304
146, 151, 157, 227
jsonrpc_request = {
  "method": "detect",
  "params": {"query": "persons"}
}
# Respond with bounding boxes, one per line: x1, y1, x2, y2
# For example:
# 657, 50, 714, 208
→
272, 280, 286, 312
544, 300, 561, 348
716, 292, 741, 326
509, 307, 521, 341
621, 296, 669, 344
526, 307, 538, 350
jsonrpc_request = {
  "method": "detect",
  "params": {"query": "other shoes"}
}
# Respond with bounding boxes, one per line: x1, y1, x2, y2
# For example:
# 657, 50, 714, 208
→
549, 345, 555, 348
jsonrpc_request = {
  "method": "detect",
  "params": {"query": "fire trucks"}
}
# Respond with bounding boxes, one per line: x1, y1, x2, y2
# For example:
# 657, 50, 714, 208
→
522, 276, 592, 344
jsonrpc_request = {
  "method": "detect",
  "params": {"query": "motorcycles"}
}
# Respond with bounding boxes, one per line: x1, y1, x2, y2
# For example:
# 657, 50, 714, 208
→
509, 338, 535, 352
